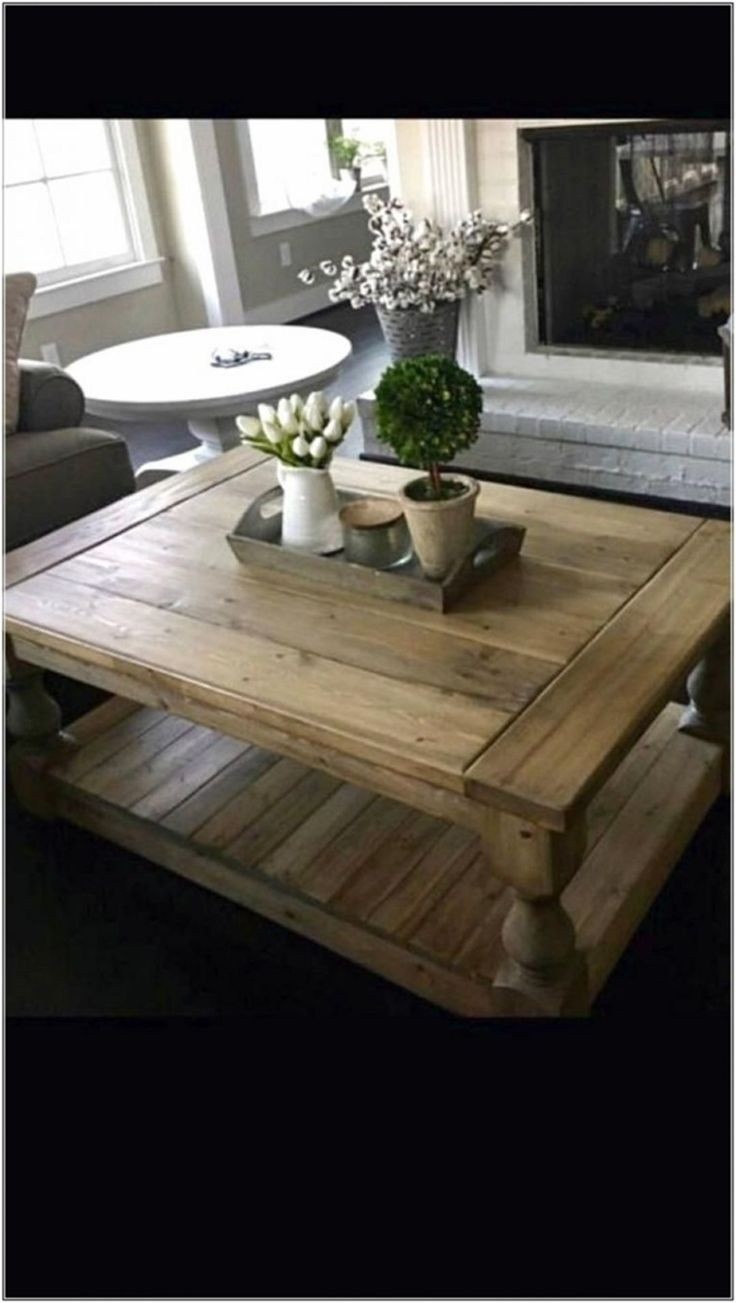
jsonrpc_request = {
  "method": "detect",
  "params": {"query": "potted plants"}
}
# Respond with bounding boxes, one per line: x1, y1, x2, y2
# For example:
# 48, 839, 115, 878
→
331, 136, 364, 191
372, 352, 486, 581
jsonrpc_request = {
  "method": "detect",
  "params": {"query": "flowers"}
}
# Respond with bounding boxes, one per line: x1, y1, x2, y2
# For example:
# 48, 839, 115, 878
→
295, 192, 536, 314
233, 390, 358, 468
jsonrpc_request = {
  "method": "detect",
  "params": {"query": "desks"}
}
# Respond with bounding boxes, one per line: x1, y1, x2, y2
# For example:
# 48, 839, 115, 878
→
3, 444, 731, 1017
59, 323, 353, 473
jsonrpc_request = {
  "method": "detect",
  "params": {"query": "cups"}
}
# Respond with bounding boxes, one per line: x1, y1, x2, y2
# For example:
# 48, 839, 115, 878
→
338, 498, 409, 569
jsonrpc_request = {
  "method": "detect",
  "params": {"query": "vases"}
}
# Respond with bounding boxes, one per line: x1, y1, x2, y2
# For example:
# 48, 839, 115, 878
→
372, 294, 462, 363
276, 459, 343, 555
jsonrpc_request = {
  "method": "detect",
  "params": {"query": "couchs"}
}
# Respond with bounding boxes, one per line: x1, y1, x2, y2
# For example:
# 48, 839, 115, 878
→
5, 358, 138, 554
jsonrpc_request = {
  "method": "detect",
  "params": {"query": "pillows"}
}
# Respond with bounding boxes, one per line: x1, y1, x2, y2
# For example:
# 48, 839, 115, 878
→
4, 270, 37, 434
